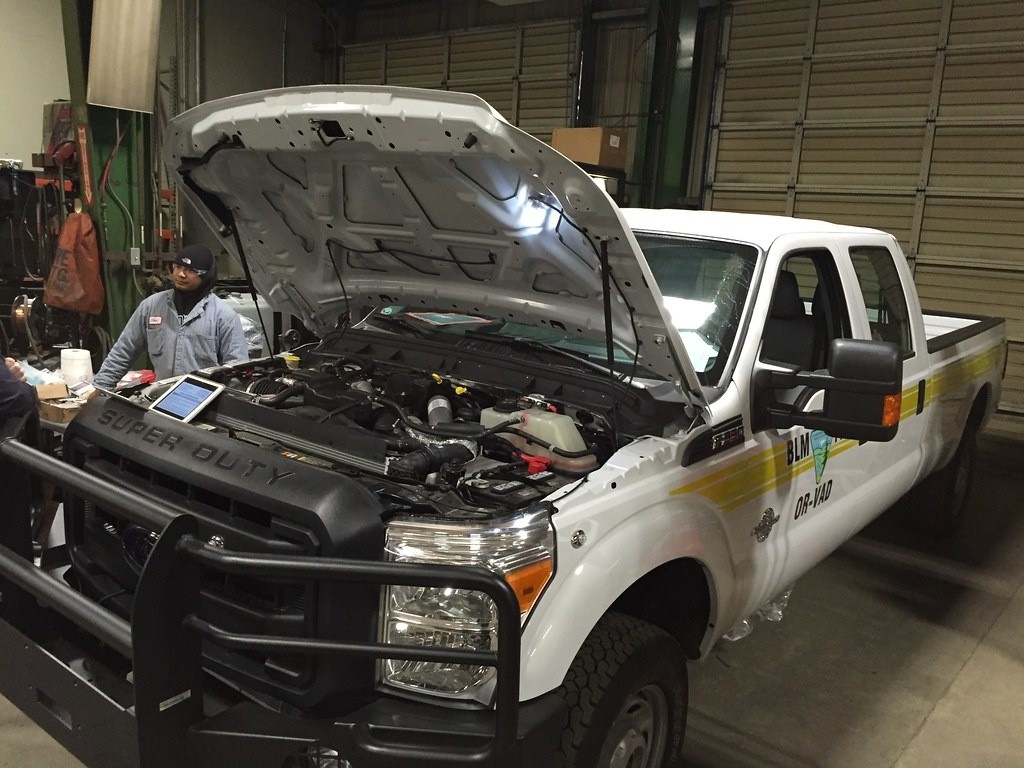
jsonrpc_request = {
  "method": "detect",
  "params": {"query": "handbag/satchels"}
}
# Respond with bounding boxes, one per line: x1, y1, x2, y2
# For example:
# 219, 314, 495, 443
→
40, 212, 105, 315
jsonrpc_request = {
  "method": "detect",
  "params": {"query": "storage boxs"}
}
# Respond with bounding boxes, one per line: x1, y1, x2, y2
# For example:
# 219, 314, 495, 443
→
551, 128, 627, 194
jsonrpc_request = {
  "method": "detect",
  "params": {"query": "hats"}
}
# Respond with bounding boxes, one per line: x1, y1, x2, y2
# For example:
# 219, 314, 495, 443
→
170, 245, 212, 273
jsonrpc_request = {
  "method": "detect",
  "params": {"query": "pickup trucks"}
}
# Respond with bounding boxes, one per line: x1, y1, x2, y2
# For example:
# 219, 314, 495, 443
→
1, 84, 1013, 768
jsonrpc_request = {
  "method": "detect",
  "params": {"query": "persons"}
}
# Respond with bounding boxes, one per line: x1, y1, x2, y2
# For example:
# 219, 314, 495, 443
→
88, 246, 248, 398
0, 356, 39, 422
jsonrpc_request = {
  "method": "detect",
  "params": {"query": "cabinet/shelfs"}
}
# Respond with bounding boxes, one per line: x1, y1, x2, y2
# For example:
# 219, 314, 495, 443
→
41, 100, 154, 376
573, 160, 628, 208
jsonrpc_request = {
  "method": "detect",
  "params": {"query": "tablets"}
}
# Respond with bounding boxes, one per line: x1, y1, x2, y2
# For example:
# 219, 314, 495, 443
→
147, 373, 226, 424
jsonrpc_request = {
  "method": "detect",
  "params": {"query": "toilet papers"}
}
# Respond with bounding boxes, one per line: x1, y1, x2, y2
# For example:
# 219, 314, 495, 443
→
60, 349, 93, 378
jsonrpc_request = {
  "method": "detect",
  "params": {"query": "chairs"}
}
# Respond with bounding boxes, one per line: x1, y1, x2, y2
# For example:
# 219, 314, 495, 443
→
724, 269, 823, 370
